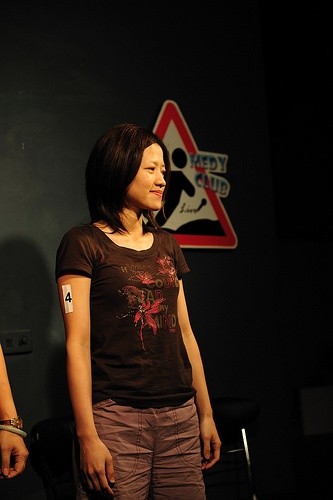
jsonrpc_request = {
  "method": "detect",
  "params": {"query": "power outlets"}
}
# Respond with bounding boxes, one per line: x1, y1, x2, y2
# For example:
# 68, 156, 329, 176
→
15, 329, 32, 353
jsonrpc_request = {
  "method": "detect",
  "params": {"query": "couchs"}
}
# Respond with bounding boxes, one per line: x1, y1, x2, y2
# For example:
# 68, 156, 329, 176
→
29, 397, 261, 500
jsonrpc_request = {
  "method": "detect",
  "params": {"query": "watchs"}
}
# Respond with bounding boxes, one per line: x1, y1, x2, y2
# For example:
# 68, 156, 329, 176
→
0, 416, 24, 430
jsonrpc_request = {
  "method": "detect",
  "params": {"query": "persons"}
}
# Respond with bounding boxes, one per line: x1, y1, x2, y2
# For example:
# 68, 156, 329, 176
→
55, 123, 222, 500
0, 343, 29, 480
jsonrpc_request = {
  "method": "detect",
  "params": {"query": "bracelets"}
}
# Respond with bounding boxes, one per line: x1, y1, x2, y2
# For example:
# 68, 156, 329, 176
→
0, 425, 27, 438
